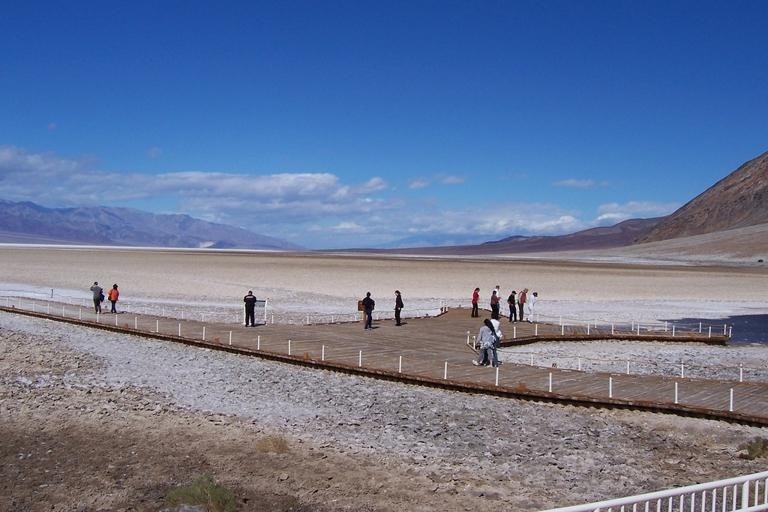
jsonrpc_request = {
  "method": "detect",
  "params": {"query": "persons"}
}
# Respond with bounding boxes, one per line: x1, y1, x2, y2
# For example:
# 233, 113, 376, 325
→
90, 282, 103, 315
491, 291, 501, 320
526, 292, 542, 324
494, 286, 502, 319
108, 284, 119, 314
362, 290, 376, 329
506, 291, 518, 322
489, 314, 500, 367
395, 290, 404, 326
518, 288, 529, 322
243, 290, 257, 326
473, 318, 494, 368
470, 287, 480, 318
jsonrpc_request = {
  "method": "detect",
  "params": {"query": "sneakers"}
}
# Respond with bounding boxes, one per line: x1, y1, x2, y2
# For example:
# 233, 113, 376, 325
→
472, 359, 497, 368
